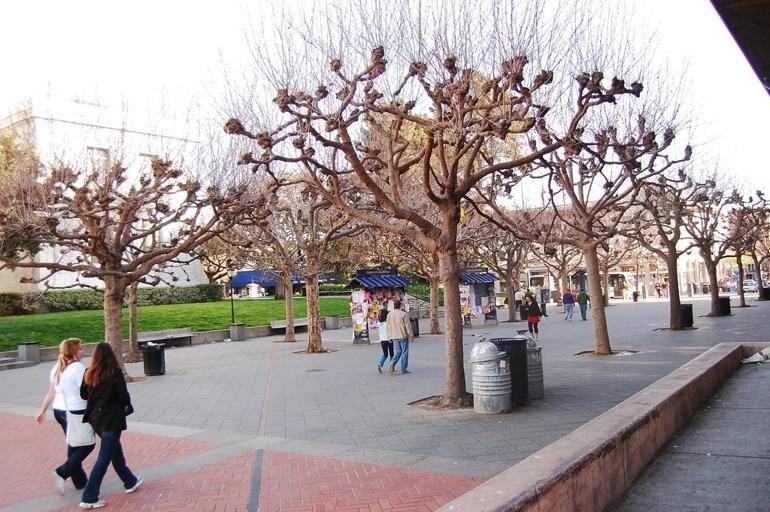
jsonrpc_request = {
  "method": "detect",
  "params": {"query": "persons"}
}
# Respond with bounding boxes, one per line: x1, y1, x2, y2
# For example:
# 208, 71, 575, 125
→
523, 295, 542, 339
79, 342, 143, 509
655, 283, 662, 298
563, 289, 575, 321
377, 309, 398, 374
35, 338, 96, 495
504, 289, 532, 311
661, 282, 668, 297
577, 289, 589, 320
387, 301, 414, 374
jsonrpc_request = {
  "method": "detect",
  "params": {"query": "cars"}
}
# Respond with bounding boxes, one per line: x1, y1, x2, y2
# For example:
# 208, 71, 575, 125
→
722, 278, 770, 294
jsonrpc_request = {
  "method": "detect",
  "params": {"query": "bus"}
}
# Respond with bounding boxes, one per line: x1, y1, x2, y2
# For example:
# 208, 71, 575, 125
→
527, 271, 634, 301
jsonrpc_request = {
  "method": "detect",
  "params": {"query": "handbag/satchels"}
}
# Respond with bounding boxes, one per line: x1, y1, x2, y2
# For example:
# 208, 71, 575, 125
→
65, 410, 96, 448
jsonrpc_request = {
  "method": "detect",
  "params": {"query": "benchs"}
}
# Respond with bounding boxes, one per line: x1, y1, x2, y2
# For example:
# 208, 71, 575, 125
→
270, 318, 308, 334
137, 326, 195, 345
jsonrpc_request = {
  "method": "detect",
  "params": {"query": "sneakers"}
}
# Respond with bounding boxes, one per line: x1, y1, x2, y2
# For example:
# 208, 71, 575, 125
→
125, 476, 145, 494
78, 499, 107, 509
377, 363, 412, 376
53, 469, 68, 496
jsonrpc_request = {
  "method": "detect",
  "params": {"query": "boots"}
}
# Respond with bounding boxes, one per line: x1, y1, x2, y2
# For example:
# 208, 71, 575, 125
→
530, 332, 539, 342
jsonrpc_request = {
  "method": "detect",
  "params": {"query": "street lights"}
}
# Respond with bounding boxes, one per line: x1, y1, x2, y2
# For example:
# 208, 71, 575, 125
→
226, 262, 237, 324
636, 255, 642, 290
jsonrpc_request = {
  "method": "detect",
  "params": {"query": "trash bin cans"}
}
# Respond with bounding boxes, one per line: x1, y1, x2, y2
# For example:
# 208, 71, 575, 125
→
703, 285, 709, 294
469, 335, 544, 414
633, 291, 639, 302
141, 342, 166, 376
